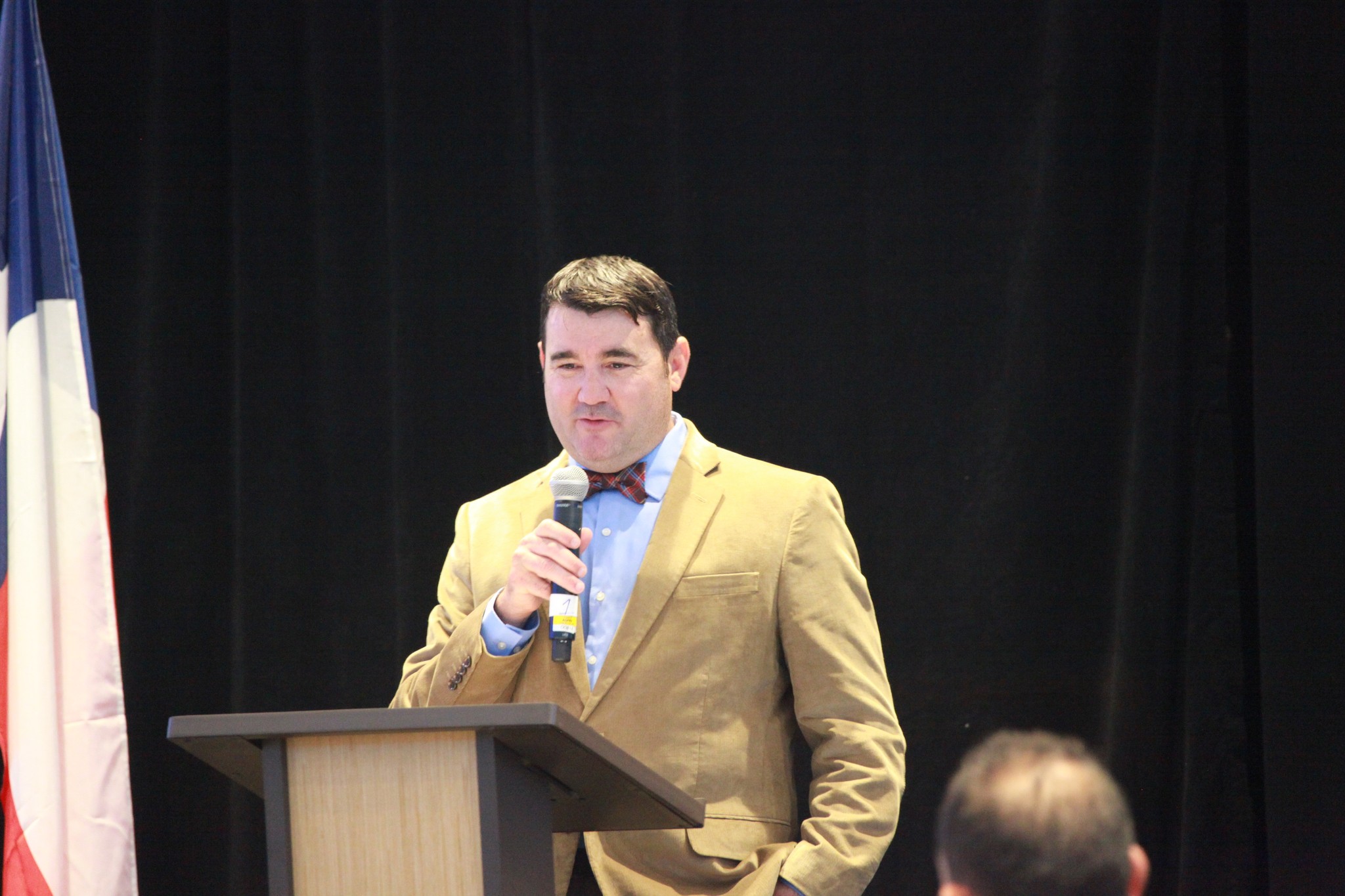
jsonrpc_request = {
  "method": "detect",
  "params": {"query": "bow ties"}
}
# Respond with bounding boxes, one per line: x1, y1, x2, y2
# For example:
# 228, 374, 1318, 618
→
581, 462, 648, 505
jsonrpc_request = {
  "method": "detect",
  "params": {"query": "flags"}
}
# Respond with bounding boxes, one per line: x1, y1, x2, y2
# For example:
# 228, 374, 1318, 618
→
0, 1, 139, 896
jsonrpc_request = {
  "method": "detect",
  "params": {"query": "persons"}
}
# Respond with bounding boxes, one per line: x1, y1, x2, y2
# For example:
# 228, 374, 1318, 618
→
384, 252, 906, 896
935, 725, 1150, 896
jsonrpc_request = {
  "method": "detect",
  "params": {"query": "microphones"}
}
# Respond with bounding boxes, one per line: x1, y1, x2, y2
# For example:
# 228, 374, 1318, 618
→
543, 466, 592, 665
